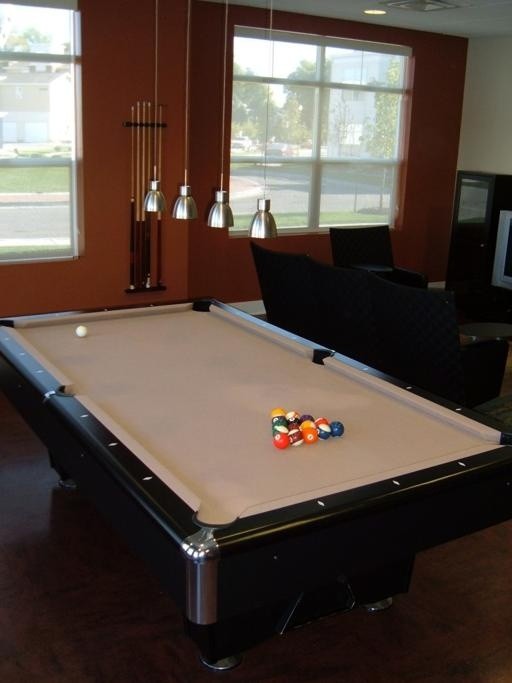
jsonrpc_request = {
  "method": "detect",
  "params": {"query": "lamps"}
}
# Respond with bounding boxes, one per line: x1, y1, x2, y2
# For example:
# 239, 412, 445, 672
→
142, 0, 281, 242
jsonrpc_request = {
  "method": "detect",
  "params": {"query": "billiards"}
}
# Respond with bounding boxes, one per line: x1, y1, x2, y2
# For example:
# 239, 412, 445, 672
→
76, 326, 87, 336
272, 408, 344, 451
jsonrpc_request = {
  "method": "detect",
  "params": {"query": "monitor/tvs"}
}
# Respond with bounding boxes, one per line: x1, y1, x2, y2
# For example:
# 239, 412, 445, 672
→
490, 209, 512, 290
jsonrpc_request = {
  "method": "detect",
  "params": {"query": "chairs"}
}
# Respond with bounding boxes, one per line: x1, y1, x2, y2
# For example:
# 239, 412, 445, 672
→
246, 244, 379, 358
328, 227, 429, 287
370, 270, 508, 411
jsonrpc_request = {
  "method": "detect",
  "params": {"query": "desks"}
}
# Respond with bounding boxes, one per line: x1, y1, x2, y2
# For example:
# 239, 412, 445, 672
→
0, 295, 512, 670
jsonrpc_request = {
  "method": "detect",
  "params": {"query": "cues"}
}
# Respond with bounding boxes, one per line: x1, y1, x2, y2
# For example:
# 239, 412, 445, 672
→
130, 102, 162, 289
